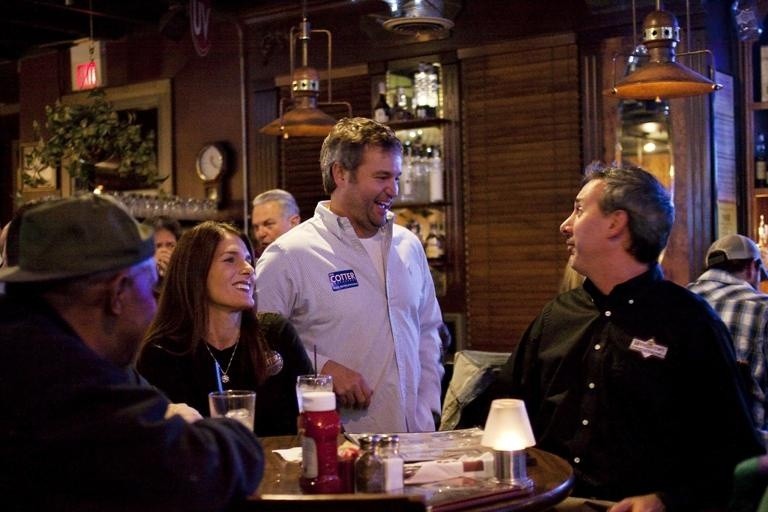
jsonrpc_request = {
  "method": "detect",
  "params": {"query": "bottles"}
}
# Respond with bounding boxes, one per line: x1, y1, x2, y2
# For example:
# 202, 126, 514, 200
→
354, 435, 382, 493
378, 435, 405, 492
426, 63, 441, 119
392, 86, 414, 121
297, 392, 342, 494
414, 61, 428, 120
373, 81, 390, 122
389, 436, 407, 461
368, 435, 381, 457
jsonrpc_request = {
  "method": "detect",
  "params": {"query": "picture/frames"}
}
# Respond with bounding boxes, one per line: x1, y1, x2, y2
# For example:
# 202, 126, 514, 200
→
17, 141, 57, 195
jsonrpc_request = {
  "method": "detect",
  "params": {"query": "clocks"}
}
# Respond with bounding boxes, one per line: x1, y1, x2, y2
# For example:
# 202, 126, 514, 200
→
196, 142, 236, 208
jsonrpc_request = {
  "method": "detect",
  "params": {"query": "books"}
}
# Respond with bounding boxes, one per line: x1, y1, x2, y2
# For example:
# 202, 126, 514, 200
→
342, 423, 500, 463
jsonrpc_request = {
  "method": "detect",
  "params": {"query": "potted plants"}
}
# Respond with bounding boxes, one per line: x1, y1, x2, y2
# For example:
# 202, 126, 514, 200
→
22, 89, 170, 222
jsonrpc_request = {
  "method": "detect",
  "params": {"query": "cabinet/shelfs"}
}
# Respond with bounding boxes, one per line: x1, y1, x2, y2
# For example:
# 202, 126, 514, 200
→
364, 52, 470, 398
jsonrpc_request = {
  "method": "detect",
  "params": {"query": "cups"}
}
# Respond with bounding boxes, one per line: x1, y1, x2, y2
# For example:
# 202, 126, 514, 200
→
295, 375, 333, 446
209, 390, 255, 434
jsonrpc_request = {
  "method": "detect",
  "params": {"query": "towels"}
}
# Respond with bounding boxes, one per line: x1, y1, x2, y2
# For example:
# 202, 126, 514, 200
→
402, 453, 499, 483
273, 446, 308, 463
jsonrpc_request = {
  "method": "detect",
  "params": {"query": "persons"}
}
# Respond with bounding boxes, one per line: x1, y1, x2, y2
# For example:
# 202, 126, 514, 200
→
682, 233, 768, 447
251, 188, 301, 249
469, 160, 738, 512
135, 222, 319, 435
143, 215, 183, 278
255, 117, 447, 433
0, 193, 266, 512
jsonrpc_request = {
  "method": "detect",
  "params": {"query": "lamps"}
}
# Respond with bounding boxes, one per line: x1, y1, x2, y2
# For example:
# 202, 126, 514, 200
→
480, 398, 536, 488
643, 121, 659, 154
259, 19, 352, 138
602, 10, 723, 101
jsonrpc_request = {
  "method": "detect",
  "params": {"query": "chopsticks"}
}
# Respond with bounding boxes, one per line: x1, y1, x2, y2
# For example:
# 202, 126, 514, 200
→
403, 452, 537, 475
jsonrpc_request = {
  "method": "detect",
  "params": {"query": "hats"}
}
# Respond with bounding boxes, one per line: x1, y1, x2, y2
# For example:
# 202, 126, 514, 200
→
1, 192, 158, 281
703, 235, 768, 281
157, 240, 158, 242
